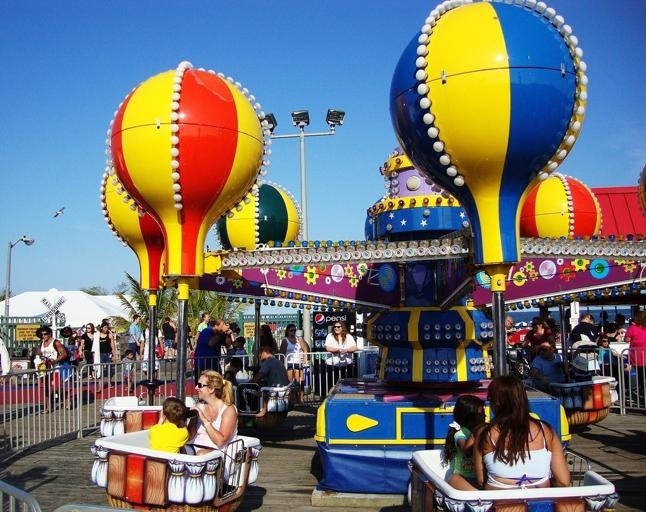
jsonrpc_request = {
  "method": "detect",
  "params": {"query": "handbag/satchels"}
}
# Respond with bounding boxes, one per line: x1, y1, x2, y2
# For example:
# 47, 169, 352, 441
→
58, 361, 72, 381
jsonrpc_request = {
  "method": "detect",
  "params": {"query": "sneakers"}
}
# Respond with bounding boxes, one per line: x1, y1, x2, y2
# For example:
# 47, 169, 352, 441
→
89, 375, 93, 380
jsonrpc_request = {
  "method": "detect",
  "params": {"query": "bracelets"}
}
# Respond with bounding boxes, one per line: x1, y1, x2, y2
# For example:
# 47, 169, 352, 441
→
204, 422, 210, 427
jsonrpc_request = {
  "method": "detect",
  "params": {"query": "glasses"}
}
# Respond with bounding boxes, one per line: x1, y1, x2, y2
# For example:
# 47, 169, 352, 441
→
41, 333, 49, 336
334, 326, 341, 328
290, 329, 296, 332
618, 332, 623, 335
197, 383, 209, 388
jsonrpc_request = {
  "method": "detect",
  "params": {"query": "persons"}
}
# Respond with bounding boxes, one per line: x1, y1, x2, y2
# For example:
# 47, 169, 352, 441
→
439, 395, 487, 491
0, 327, 11, 386
180, 369, 238, 456
472, 375, 571, 490
148, 397, 190, 453
280, 324, 308, 404
324, 321, 357, 392
36, 312, 249, 415
504, 311, 646, 403
252, 324, 278, 353
238, 345, 290, 413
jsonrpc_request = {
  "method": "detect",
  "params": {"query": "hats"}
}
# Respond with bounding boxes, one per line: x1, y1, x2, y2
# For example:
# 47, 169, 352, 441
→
36, 326, 52, 337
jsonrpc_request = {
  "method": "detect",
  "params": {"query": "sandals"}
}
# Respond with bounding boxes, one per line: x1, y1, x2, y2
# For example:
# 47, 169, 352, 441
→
96, 385, 114, 392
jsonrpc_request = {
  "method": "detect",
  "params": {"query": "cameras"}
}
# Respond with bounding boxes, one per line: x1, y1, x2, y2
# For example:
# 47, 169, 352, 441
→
229, 322, 241, 335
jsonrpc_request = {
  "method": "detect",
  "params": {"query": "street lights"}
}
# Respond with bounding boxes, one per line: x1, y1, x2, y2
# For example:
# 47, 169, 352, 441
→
261, 108, 345, 354
4, 237, 35, 321
